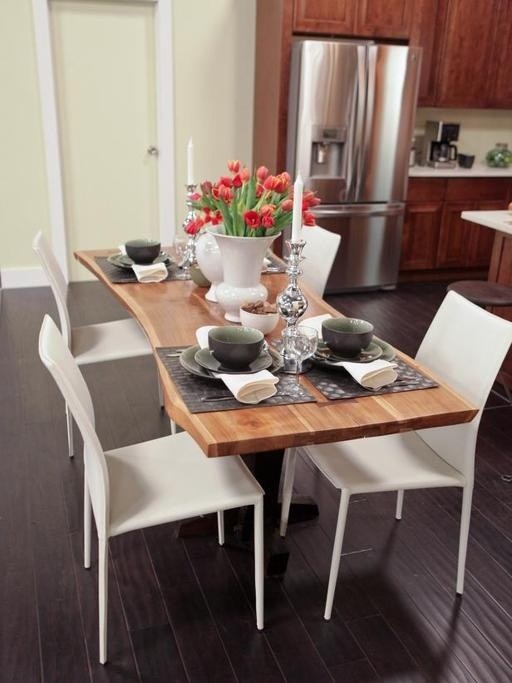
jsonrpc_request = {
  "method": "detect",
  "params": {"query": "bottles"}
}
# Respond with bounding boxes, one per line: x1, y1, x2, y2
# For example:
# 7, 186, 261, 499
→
485, 143, 512, 168
408, 138, 416, 167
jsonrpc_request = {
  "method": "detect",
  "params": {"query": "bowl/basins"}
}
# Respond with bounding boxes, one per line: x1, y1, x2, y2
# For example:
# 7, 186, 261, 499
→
321, 318, 374, 358
189, 264, 211, 288
239, 306, 280, 336
208, 326, 264, 372
124, 239, 161, 265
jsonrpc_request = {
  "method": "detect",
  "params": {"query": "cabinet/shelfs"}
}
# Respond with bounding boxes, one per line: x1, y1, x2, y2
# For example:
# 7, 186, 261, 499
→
398, 177, 511, 283
435, 0, 512, 109
292, 0, 413, 41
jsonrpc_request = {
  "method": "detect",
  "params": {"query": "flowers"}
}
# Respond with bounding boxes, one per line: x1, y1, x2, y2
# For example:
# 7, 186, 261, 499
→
184, 159, 321, 236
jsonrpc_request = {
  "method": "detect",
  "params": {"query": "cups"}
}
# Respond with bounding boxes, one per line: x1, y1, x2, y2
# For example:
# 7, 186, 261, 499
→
458, 153, 475, 169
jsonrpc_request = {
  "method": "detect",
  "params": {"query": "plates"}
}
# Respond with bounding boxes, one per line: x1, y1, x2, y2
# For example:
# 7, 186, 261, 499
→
178, 344, 281, 379
107, 252, 175, 268
310, 337, 396, 364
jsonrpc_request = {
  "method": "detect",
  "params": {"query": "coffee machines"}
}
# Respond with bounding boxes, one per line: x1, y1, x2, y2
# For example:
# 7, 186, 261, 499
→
420, 121, 461, 169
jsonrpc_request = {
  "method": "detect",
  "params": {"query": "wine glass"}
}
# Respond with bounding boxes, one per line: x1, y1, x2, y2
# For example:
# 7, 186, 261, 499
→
281, 325, 319, 398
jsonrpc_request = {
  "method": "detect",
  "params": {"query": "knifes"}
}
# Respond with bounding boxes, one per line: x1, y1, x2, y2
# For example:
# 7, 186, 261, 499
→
201, 394, 290, 402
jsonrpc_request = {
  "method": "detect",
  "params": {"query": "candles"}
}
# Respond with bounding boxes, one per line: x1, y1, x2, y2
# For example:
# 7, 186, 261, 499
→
291, 171, 304, 243
186, 136, 196, 184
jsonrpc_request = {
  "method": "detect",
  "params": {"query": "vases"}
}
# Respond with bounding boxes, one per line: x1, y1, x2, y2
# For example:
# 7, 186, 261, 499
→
205, 224, 282, 324
196, 226, 221, 303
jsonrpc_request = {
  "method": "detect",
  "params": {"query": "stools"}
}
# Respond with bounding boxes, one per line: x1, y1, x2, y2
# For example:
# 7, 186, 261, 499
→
446, 280, 512, 313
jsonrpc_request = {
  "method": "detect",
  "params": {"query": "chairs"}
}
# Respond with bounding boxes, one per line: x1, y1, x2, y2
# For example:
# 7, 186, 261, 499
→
279, 290, 512, 621
31, 229, 177, 459
300, 225, 341, 298
38, 314, 267, 665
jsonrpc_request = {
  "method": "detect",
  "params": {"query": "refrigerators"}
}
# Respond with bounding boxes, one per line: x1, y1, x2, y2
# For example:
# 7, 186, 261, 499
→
284, 40, 423, 294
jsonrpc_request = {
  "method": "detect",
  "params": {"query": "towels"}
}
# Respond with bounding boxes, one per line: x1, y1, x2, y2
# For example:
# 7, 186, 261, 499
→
119, 243, 168, 282
299, 313, 398, 388
196, 327, 279, 405
